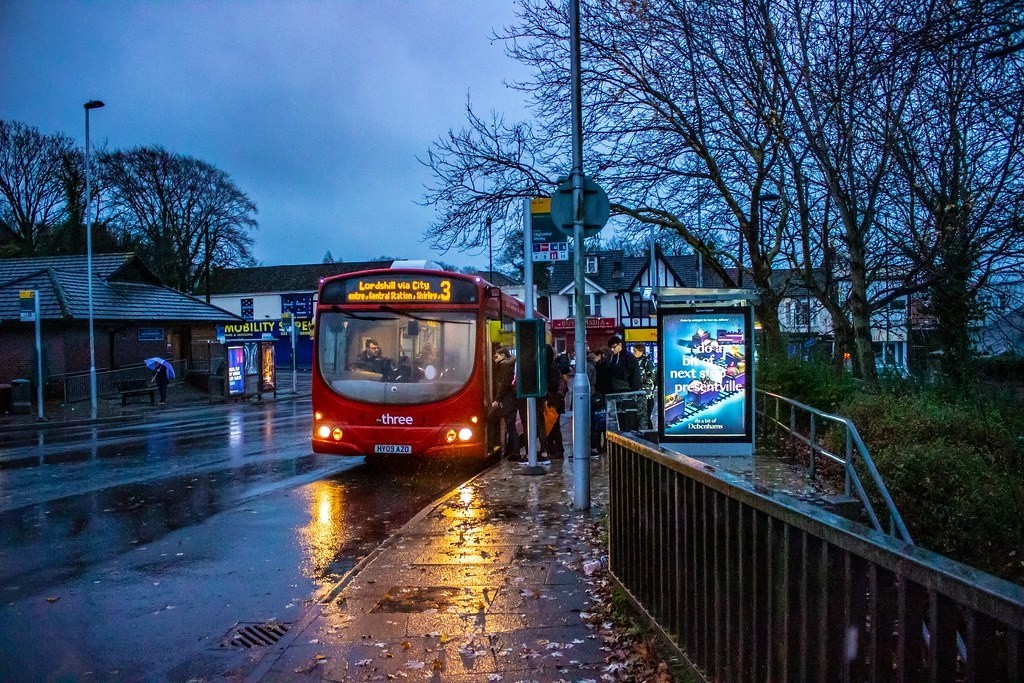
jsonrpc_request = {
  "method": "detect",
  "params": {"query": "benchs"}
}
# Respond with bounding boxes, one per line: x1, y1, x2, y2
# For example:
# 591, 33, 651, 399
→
119, 378, 159, 408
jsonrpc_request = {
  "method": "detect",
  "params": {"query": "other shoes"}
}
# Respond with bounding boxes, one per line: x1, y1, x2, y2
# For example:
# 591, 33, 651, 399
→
158, 400, 166, 405
505, 447, 565, 463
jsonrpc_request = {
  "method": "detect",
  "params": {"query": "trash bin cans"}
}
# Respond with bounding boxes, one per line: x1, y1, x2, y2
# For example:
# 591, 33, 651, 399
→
11, 379, 32, 414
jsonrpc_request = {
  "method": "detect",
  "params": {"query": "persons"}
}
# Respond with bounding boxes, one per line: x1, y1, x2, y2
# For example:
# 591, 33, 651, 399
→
421, 336, 655, 465
152, 361, 168, 405
356, 338, 392, 382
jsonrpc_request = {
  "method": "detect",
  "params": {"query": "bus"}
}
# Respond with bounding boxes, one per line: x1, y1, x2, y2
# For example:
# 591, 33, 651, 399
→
314, 259, 547, 459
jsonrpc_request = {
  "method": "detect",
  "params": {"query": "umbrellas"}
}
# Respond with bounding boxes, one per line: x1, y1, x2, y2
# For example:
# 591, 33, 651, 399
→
143, 357, 175, 381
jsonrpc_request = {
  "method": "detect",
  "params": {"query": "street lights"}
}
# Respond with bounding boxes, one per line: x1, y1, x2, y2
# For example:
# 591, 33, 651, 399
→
83, 98, 105, 422
